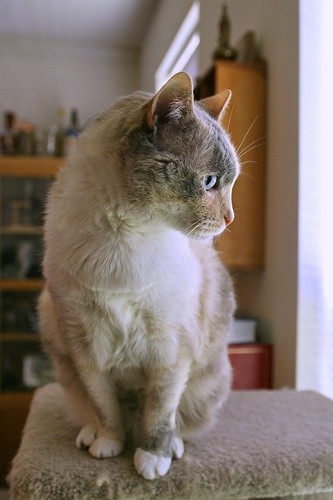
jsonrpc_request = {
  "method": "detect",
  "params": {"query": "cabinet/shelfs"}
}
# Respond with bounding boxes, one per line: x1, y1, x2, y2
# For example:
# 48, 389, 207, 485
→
0, 158, 73, 489
229, 342, 272, 390
194, 60, 267, 271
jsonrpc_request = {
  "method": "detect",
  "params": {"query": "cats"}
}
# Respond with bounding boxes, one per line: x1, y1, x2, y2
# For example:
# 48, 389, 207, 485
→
34, 70, 266, 480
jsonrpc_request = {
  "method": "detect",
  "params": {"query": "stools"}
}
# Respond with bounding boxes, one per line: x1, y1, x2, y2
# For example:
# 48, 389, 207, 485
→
8, 382, 333, 500
